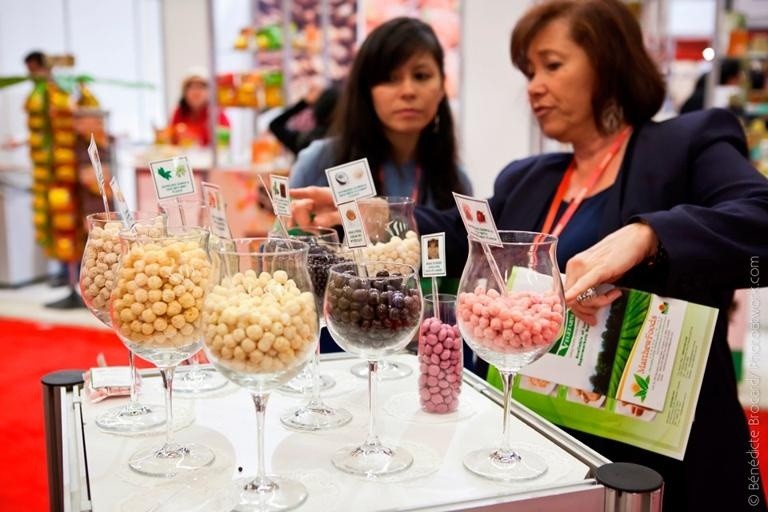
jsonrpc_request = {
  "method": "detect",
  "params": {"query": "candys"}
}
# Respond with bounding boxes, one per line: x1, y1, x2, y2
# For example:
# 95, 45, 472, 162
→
325, 267, 422, 347
350, 233, 423, 276
108, 229, 210, 348
204, 268, 318, 371
79, 208, 167, 329
454, 286, 565, 355
417, 316, 462, 415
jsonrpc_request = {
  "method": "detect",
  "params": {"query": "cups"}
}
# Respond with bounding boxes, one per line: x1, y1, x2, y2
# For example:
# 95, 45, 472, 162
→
156, 201, 240, 391
80, 212, 168, 432
343, 196, 420, 381
323, 261, 423, 476
456, 231, 566, 480
109, 228, 215, 476
200, 237, 319, 512
272, 240, 354, 432
263, 227, 339, 395
418, 294, 463, 415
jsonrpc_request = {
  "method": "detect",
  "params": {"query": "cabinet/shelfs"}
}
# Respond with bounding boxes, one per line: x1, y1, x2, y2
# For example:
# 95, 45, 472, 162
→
133, 165, 291, 272
41, 338, 665, 511
250, 2, 466, 166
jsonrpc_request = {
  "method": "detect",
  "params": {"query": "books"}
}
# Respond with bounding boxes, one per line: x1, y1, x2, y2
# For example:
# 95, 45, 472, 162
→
485, 265, 719, 461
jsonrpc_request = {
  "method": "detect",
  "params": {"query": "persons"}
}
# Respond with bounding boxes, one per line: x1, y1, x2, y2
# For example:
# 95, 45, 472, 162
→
162, 73, 230, 147
259, 16, 477, 367
464, 1, 768, 512
268, 80, 341, 163
715, 56, 747, 109
24, 51, 80, 288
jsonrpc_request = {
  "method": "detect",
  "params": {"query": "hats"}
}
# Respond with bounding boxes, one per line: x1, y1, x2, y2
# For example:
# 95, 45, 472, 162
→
184, 75, 209, 88
